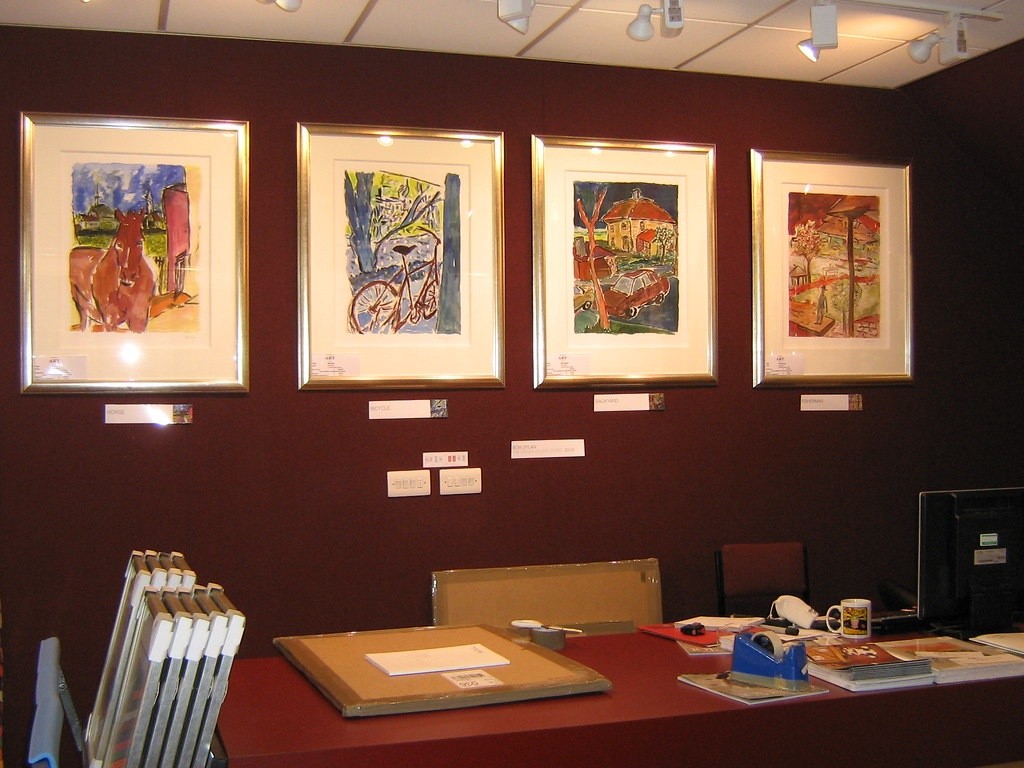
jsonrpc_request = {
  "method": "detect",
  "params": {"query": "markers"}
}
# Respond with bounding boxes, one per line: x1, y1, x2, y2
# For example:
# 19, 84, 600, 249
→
758, 624, 799, 635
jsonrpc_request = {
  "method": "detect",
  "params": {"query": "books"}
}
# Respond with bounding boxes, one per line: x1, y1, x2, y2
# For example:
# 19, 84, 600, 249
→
635, 615, 851, 656
806, 633, 1024, 692
677, 670, 830, 705
365, 643, 511, 677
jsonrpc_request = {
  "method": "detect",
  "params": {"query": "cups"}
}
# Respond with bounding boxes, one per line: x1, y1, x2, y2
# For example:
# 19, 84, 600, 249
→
826, 598, 872, 640
529, 628, 566, 650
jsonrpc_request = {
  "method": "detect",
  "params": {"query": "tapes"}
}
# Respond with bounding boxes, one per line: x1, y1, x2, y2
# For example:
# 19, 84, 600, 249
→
751, 631, 784, 658
528, 628, 567, 649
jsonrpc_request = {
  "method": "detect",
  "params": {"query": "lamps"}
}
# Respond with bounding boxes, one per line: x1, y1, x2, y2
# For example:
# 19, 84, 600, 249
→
907, 12, 969, 67
256, 0, 302, 12
626, 0, 684, 43
497, 0, 536, 35
797, 0, 839, 63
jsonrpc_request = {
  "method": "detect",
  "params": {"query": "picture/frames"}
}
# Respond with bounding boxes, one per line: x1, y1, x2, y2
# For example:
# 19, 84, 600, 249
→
750, 147, 919, 389
272, 624, 613, 717
297, 121, 504, 387
84, 550, 246, 768
19, 110, 250, 394
429, 558, 663, 635
531, 133, 720, 387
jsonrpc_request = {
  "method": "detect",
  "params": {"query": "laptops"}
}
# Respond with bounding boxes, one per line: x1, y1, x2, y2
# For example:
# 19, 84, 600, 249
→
810, 487, 1024, 633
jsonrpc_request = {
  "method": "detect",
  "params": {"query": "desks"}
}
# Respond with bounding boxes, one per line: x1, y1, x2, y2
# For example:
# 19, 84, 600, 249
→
205, 613, 1024, 768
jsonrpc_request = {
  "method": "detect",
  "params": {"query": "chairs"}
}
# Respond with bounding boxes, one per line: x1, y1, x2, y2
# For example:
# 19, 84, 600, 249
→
715, 541, 814, 621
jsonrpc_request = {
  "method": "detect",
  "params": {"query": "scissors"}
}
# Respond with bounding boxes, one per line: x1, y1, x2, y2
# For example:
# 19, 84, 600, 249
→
511, 620, 583, 633
